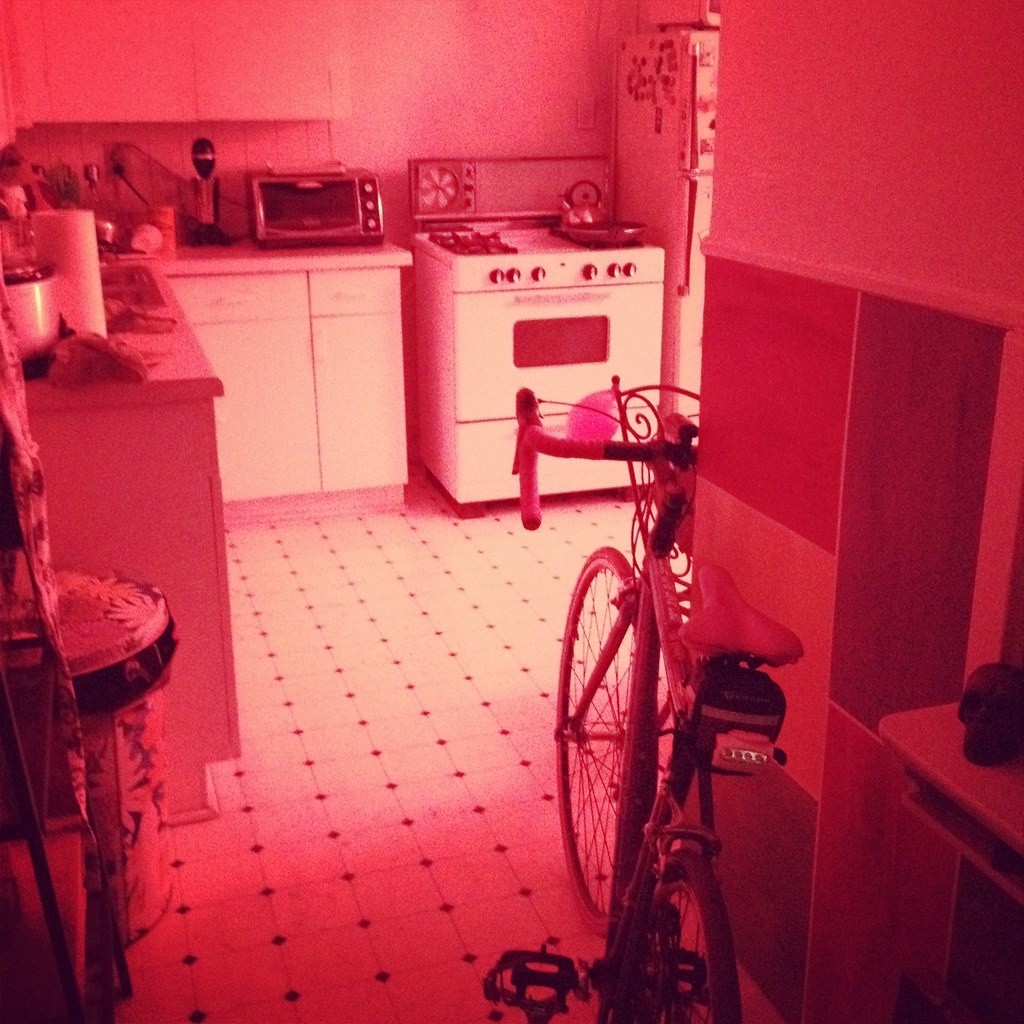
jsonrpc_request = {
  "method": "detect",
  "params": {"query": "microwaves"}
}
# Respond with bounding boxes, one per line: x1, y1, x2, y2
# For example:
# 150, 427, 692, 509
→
248, 168, 385, 250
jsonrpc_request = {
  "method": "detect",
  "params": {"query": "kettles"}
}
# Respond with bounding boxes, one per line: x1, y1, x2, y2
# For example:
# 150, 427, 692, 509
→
560, 178, 608, 229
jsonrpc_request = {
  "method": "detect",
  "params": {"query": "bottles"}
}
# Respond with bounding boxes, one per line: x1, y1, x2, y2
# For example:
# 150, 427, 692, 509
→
4, 262, 64, 354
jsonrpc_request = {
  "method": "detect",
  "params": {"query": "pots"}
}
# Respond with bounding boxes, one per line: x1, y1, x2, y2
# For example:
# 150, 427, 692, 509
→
564, 221, 649, 244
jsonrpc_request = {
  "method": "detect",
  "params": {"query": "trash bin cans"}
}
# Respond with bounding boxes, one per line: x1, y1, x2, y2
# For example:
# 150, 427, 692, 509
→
50, 570, 175, 952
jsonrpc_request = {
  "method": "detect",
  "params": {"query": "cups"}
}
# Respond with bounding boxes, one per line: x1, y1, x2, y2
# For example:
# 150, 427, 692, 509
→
146, 204, 175, 253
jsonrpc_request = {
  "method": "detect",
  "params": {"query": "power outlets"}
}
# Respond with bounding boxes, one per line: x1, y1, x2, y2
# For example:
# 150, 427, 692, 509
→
103, 144, 131, 181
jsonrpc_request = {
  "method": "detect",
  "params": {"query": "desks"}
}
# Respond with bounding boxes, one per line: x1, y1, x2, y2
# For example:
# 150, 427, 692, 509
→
879, 701, 1024, 1024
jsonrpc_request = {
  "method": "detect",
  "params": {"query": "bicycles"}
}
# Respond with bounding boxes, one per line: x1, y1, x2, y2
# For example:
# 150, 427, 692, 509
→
481, 376, 803, 1024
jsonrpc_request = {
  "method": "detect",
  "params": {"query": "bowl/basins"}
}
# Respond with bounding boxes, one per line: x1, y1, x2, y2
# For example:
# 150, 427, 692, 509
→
132, 224, 163, 252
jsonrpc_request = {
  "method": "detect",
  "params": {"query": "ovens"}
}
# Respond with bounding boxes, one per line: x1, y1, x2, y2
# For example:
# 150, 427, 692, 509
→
415, 280, 663, 521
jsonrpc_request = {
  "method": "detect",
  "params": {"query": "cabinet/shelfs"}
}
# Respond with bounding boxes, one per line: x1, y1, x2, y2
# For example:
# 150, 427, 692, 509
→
1, 0, 350, 148
30, 396, 244, 825
308, 268, 410, 492
0, 443, 133, 1024
168, 272, 321, 503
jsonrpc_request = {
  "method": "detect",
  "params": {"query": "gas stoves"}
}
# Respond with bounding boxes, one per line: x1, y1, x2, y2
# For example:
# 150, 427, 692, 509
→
407, 152, 665, 294
